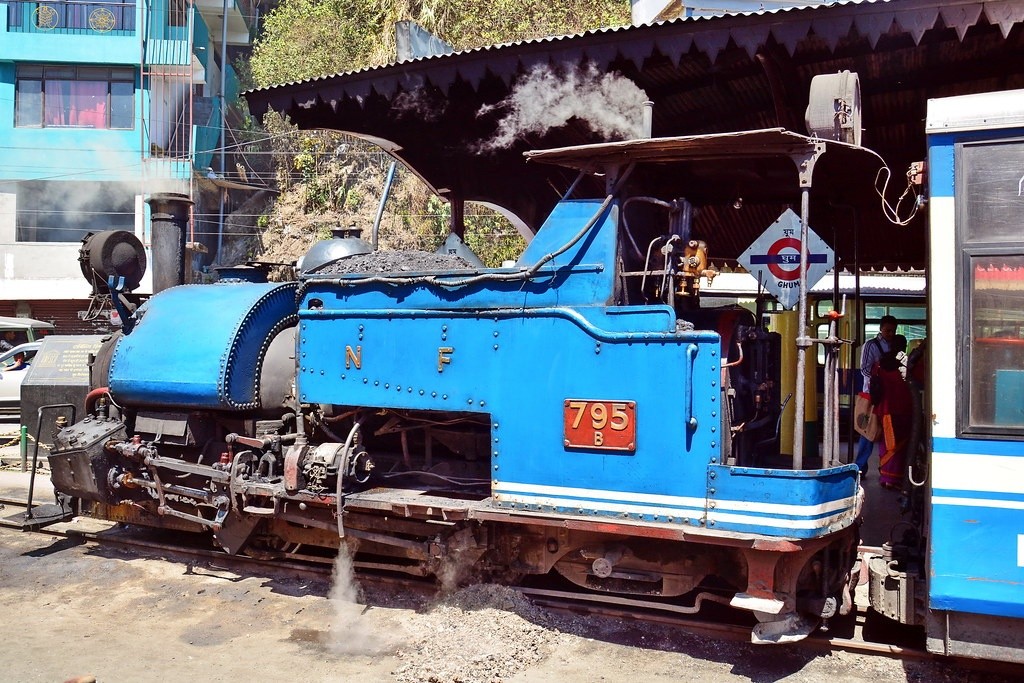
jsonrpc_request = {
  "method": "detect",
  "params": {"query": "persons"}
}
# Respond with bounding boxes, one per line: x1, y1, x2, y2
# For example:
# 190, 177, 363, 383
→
871, 333, 911, 490
0, 331, 31, 370
856, 315, 898, 479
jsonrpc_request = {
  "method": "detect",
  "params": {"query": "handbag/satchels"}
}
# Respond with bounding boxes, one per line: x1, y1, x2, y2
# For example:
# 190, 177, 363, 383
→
854, 391, 883, 442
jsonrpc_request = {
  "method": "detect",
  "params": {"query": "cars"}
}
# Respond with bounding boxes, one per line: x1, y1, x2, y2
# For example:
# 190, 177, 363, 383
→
0, 316, 56, 415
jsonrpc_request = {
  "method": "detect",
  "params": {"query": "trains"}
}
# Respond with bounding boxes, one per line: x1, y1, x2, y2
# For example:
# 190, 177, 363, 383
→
44, 87, 1024, 666
